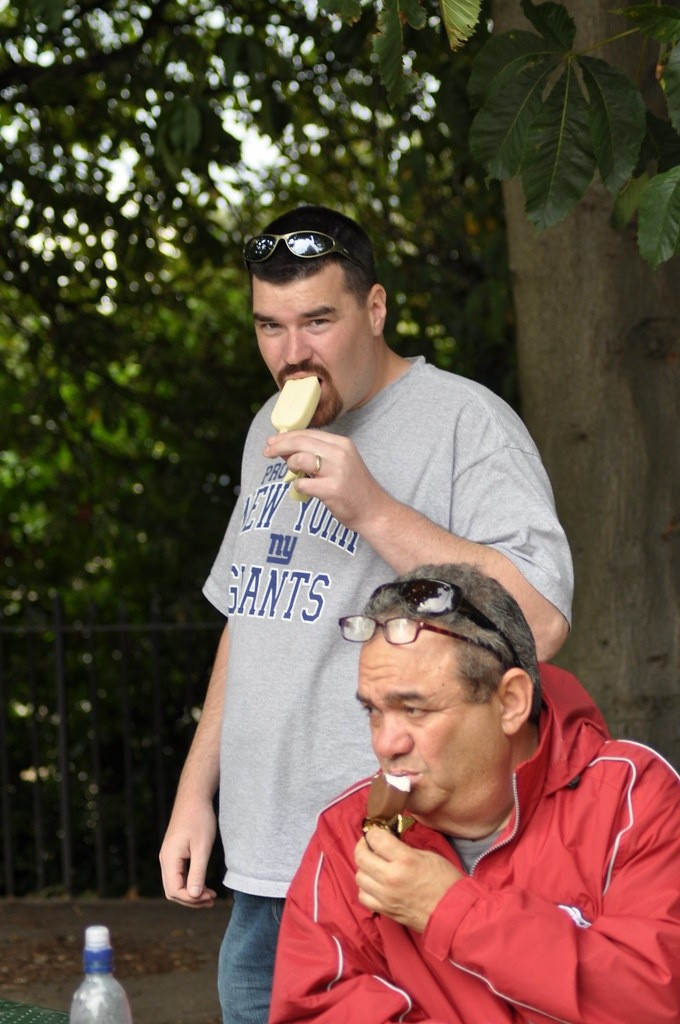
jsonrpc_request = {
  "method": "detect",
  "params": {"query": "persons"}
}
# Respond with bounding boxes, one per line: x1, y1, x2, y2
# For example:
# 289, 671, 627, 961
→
265, 562, 680, 1024
158, 206, 574, 1024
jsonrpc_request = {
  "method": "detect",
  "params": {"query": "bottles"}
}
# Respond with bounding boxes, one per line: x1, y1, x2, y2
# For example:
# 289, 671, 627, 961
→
70, 925, 131, 1024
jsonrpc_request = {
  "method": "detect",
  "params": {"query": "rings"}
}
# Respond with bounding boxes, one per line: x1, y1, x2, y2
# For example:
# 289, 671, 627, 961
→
314, 455, 321, 476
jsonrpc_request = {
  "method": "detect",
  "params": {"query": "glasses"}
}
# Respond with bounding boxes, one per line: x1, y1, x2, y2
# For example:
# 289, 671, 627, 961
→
242, 229, 379, 289
365, 577, 524, 670
338, 615, 511, 677
